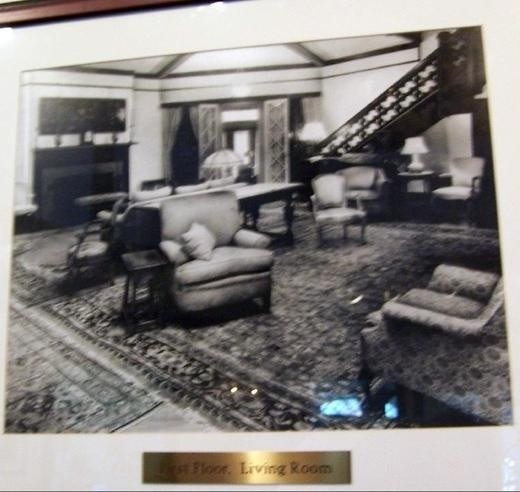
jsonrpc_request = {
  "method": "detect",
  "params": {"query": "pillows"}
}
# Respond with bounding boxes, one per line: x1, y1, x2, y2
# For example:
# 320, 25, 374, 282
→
182, 222, 216, 262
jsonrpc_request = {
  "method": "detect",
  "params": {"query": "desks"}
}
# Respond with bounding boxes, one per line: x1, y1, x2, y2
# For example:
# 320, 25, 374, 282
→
397, 170, 433, 216
119, 248, 173, 333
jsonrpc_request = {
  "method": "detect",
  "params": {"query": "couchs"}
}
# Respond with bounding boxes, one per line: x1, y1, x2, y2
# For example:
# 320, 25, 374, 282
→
359, 264, 513, 424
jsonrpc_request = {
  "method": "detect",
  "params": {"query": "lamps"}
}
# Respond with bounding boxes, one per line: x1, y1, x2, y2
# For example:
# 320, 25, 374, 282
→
399, 136, 429, 171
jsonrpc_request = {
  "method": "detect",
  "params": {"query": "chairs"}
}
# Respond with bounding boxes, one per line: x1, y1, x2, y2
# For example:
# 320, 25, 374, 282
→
310, 173, 368, 243
335, 166, 399, 222
122, 189, 273, 319
65, 193, 127, 286
430, 156, 486, 221
